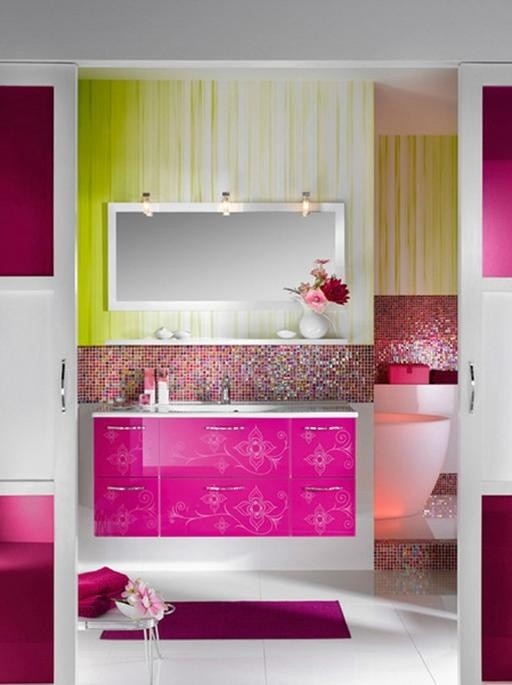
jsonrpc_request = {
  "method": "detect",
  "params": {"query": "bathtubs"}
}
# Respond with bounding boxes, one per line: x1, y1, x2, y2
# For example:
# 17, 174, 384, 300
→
374, 411, 452, 519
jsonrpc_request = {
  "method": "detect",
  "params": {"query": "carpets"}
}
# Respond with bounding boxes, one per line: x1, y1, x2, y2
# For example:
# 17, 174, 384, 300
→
100, 599, 350, 642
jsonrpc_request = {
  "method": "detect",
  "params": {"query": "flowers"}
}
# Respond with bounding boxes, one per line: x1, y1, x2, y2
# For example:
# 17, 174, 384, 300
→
284, 259, 349, 314
115, 579, 162, 621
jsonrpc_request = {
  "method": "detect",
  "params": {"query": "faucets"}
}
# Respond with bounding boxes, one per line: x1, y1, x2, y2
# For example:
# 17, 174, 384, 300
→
221, 376, 230, 403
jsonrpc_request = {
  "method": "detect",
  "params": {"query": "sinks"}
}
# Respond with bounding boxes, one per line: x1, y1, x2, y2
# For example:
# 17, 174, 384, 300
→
167, 404, 285, 412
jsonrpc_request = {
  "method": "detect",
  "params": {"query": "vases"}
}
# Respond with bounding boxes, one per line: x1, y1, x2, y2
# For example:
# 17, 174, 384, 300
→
298, 305, 328, 338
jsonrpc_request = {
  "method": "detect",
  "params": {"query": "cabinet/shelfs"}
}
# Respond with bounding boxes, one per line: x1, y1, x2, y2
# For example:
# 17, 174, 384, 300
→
91, 417, 358, 537
